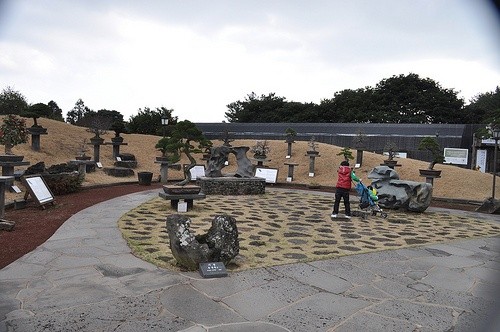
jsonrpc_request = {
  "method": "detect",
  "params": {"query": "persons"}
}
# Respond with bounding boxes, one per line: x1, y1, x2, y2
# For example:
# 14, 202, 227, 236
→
367, 185, 382, 211
330, 161, 362, 219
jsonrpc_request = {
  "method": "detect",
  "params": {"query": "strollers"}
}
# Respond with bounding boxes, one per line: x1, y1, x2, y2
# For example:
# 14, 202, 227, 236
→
351, 171, 387, 219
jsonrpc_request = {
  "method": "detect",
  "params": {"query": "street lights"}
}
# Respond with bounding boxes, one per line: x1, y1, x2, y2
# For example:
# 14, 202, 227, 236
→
492, 126, 500, 202
161, 113, 169, 157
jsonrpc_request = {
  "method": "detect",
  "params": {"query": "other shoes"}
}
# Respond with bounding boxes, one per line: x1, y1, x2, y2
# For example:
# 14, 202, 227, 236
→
331, 214, 338, 218
345, 215, 351, 218
